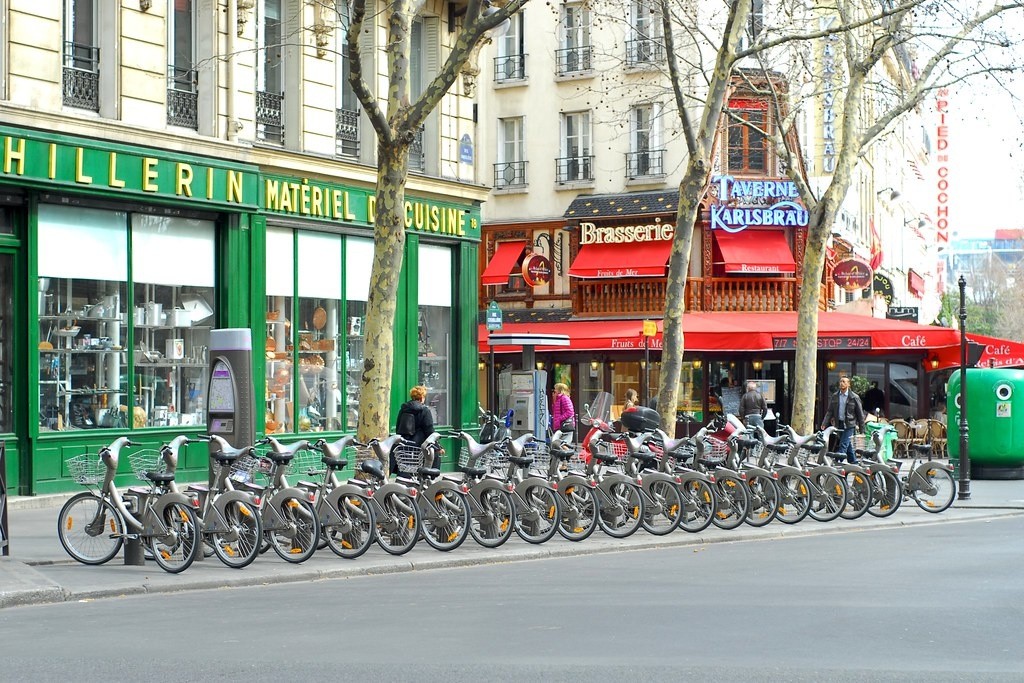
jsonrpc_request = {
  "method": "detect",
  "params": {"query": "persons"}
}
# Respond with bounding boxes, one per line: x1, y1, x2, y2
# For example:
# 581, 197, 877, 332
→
551, 383, 576, 448
620, 388, 638, 433
820, 375, 866, 467
934, 400, 947, 425
739, 381, 767, 442
395, 385, 445, 479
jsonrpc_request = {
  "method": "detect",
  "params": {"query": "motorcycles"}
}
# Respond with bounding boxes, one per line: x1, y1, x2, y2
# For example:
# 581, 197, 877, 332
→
622, 405, 750, 470
580, 391, 660, 463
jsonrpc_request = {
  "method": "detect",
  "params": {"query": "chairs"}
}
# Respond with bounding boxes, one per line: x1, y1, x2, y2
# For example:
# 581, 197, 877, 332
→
888, 417, 948, 459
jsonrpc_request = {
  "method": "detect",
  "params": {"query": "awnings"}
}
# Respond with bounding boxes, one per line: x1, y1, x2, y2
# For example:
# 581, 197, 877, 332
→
477, 311, 972, 373
960, 332, 1024, 371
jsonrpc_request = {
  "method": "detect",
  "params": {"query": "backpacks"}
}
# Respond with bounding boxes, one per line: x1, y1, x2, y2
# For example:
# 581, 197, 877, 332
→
397, 407, 424, 435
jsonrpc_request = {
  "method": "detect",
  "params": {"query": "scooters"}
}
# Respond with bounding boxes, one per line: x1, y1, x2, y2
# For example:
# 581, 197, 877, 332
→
54, 415, 963, 573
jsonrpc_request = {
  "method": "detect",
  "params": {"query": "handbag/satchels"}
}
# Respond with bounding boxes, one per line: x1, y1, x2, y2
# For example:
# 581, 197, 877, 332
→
561, 417, 575, 431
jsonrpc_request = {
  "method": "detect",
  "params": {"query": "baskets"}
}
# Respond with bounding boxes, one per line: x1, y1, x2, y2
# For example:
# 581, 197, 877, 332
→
66, 454, 108, 484
459, 446, 489, 471
346, 443, 378, 470
210, 449, 260, 482
298, 445, 328, 474
256, 446, 294, 475
393, 445, 423, 473
849, 434, 877, 453
486, 436, 811, 468
127, 449, 165, 480
